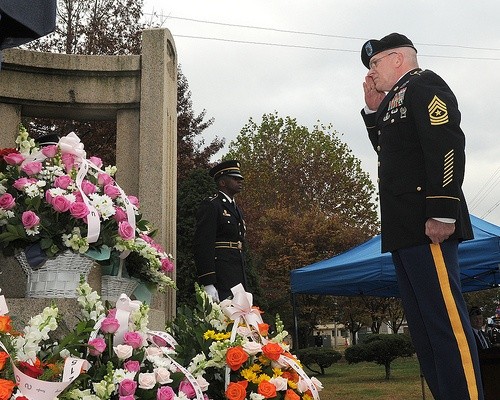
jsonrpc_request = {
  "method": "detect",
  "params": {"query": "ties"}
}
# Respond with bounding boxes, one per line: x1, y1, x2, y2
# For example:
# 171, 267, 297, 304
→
478, 331, 488, 349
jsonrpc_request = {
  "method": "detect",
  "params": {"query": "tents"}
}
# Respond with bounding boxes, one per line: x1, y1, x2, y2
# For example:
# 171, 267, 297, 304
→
287, 211, 499, 363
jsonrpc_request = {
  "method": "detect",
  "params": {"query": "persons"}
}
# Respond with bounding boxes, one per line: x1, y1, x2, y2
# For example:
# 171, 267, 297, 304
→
467, 305, 492, 351
193, 158, 251, 309
485, 316, 500, 346
360, 31, 488, 400
315, 332, 324, 348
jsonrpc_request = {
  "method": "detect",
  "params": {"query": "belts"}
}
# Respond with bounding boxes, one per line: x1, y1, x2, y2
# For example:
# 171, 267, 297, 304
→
215, 241, 242, 249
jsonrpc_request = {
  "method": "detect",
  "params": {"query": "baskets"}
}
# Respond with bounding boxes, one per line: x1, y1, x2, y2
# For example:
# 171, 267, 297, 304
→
101, 275, 140, 301
15, 251, 96, 299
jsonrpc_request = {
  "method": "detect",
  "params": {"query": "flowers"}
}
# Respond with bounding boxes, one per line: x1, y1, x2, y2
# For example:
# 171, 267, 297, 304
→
0, 122, 324, 400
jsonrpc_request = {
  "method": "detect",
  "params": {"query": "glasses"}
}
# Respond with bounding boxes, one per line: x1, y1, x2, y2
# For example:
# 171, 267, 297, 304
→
368, 52, 397, 69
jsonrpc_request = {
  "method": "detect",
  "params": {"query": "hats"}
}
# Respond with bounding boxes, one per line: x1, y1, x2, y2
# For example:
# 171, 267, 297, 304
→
469, 306, 482, 316
209, 160, 243, 179
361, 33, 417, 70
34, 134, 60, 146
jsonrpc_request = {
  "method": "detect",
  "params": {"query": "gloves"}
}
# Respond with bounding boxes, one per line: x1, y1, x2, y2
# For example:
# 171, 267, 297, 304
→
202, 284, 220, 303
